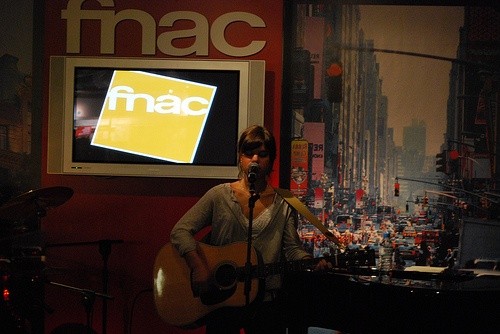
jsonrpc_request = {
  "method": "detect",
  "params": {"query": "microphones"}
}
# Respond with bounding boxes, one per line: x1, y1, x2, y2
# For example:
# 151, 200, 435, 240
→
248, 162, 259, 180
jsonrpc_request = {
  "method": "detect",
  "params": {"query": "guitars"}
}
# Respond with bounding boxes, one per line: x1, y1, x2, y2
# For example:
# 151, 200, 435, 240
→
154, 237, 376, 326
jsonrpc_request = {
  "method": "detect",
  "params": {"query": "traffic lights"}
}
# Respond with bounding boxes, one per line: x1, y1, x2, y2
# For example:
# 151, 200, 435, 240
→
449, 150, 459, 171
327, 61, 342, 104
394, 183, 399, 197
435, 150, 448, 175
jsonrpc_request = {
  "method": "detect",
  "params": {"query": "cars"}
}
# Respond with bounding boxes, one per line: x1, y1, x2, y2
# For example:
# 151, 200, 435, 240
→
299, 205, 441, 267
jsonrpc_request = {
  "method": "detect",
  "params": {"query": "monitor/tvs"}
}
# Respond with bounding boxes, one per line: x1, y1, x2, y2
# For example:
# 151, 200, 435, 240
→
46, 55, 268, 180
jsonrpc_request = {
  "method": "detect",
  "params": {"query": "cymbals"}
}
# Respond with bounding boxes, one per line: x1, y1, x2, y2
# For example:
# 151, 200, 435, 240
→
0, 186, 74, 221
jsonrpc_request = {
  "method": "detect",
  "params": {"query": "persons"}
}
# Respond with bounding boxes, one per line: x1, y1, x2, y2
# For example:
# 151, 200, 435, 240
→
170, 125, 328, 334
